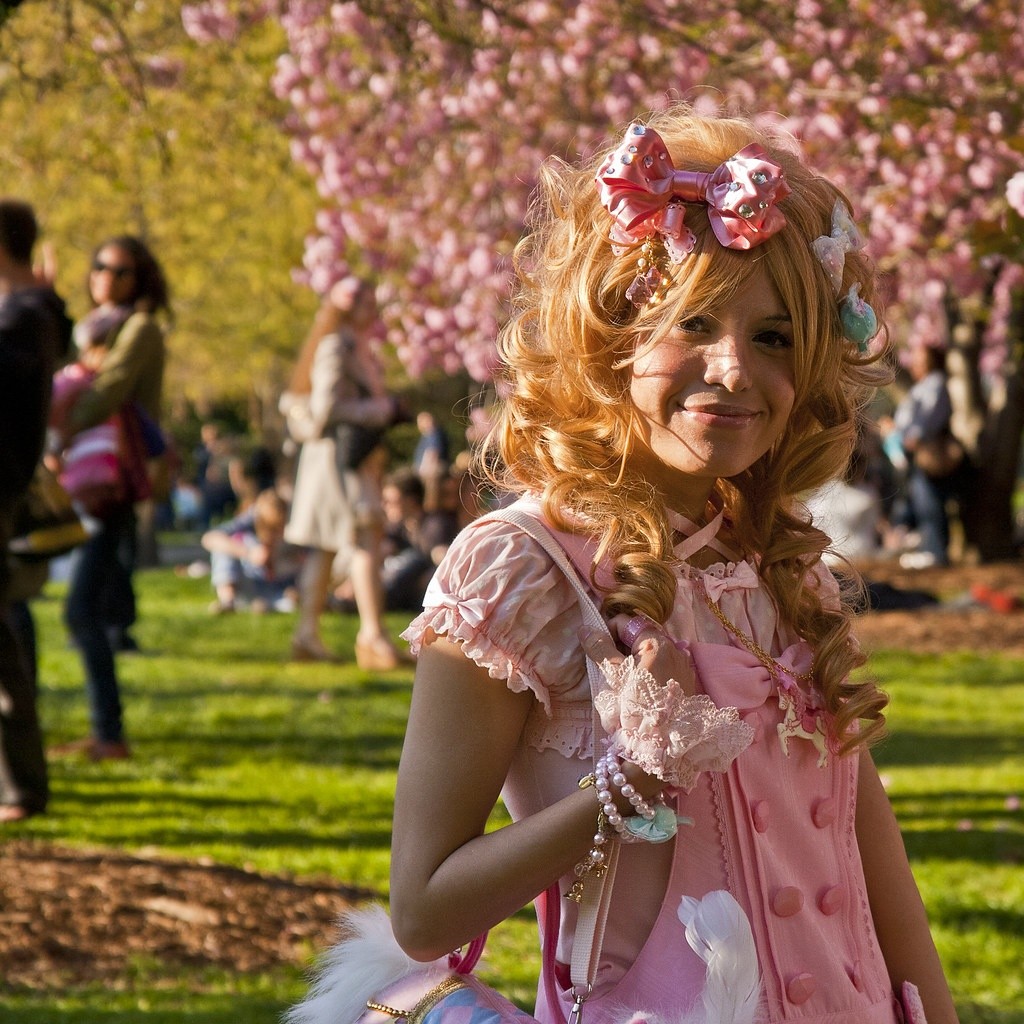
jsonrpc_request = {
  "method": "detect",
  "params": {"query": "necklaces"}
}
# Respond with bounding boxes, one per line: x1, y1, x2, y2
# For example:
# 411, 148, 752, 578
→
692, 568, 829, 768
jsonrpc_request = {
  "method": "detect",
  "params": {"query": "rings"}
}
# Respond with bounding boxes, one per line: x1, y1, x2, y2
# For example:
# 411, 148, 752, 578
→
618, 615, 652, 649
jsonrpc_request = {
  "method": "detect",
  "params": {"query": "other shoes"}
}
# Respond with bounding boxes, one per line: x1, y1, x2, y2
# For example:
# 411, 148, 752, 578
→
293, 641, 334, 660
48, 742, 127, 762
353, 644, 413, 669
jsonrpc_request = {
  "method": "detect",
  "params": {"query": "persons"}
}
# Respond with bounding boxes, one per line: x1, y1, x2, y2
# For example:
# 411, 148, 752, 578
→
389, 116, 960, 1024
0, 199, 170, 824
170, 276, 527, 672
794, 341, 1024, 569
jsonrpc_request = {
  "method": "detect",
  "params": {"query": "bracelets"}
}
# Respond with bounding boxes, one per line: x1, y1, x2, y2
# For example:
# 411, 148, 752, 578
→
562, 749, 664, 903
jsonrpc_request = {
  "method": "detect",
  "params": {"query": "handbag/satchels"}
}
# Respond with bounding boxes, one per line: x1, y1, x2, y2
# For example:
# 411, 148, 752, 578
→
282, 507, 632, 1024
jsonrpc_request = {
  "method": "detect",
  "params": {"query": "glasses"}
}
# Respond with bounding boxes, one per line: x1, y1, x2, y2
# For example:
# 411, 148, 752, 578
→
93, 259, 137, 279
